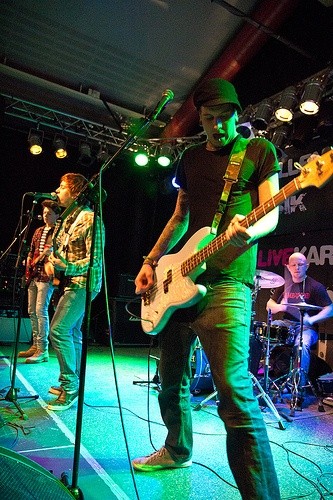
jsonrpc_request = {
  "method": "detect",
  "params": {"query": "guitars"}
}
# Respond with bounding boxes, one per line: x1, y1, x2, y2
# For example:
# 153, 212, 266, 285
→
51, 215, 66, 288
139, 146, 333, 336
18, 242, 53, 290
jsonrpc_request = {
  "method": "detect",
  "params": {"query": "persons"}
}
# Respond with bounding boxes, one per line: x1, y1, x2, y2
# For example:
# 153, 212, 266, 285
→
265, 252, 333, 412
47, 172, 106, 410
18, 200, 62, 364
133, 78, 281, 500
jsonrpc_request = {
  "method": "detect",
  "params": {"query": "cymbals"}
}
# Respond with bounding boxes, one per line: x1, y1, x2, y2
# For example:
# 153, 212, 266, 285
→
283, 300, 325, 310
254, 269, 286, 289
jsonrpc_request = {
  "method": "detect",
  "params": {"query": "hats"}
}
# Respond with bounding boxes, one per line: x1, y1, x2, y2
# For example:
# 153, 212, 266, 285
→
193, 79, 243, 113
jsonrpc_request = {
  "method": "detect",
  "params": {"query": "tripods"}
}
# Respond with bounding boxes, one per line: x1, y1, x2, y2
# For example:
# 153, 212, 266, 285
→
132, 355, 162, 391
194, 277, 326, 429
0, 199, 39, 421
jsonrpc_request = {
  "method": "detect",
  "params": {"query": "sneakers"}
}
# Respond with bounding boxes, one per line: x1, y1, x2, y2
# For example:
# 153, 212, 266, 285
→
47, 390, 79, 410
26, 349, 50, 363
132, 445, 192, 471
49, 386, 63, 395
19, 345, 37, 357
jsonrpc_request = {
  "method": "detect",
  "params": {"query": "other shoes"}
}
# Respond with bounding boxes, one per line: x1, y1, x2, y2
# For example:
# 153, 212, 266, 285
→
290, 396, 302, 406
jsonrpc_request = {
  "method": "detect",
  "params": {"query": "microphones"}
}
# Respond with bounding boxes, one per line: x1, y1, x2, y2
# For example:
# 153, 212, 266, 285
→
24, 214, 42, 221
150, 89, 174, 124
27, 192, 57, 200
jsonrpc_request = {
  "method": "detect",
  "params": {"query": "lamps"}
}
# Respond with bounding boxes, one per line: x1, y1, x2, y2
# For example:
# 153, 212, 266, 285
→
26, 128, 44, 154
254, 99, 275, 128
270, 126, 290, 152
288, 116, 313, 150
78, 140, 93, 166
299, 76, 324, 116
275, 86, 300, 120
135, 142, 151, 166
237, 105, 254, 139
52, 132, 68, 158
313, 100, 333, 142
157, 143, 174, 166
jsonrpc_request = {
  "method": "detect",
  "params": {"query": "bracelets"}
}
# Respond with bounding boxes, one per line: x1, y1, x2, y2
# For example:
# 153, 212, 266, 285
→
142, 255, 156, 269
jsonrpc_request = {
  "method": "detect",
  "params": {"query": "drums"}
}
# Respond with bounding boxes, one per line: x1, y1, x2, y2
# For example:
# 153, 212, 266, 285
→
190, 337, 259, 396
254, 322, 294, 345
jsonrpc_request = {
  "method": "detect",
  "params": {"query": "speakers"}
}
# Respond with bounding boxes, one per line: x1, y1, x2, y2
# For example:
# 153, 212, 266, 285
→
0, 445, 76, 500
111, 298, 154, 348
